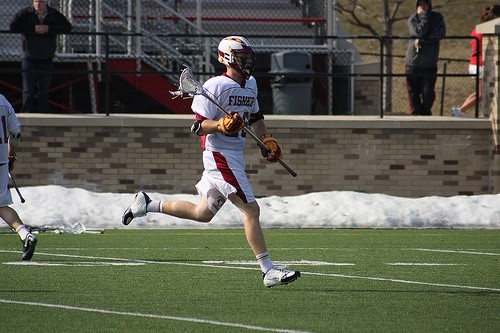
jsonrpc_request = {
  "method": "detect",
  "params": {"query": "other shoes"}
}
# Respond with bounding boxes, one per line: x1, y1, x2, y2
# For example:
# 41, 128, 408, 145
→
451, 106, 465, 116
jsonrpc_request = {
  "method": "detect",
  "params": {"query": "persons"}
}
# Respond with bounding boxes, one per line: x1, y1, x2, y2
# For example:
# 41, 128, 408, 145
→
0, 93, 37, 261
122, 36, 301, 289
492, 5, 500, 19
405, 0, 447, 116
451, 6, 493, 117
10, 1, 71, 113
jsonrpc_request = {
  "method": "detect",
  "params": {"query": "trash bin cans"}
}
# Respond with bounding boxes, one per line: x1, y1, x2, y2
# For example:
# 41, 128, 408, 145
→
268, 49, 315, 115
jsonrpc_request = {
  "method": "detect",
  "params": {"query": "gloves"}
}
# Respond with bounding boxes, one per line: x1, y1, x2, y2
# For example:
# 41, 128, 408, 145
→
257, 133, 281, 162
217, 111, 244, 135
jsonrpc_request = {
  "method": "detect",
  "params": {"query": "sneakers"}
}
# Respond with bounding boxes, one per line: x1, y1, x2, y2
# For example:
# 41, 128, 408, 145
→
20, 232, 37, 260
261, 265, 301, 288
122, 190, 152, 225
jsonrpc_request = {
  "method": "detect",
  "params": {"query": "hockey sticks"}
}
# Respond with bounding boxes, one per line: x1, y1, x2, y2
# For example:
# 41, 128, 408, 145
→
168, 64, 297, 178
8, 171, 27, 204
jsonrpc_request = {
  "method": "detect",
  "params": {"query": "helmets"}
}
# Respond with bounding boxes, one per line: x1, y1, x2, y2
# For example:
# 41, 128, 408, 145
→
217, 35, 256, 79
